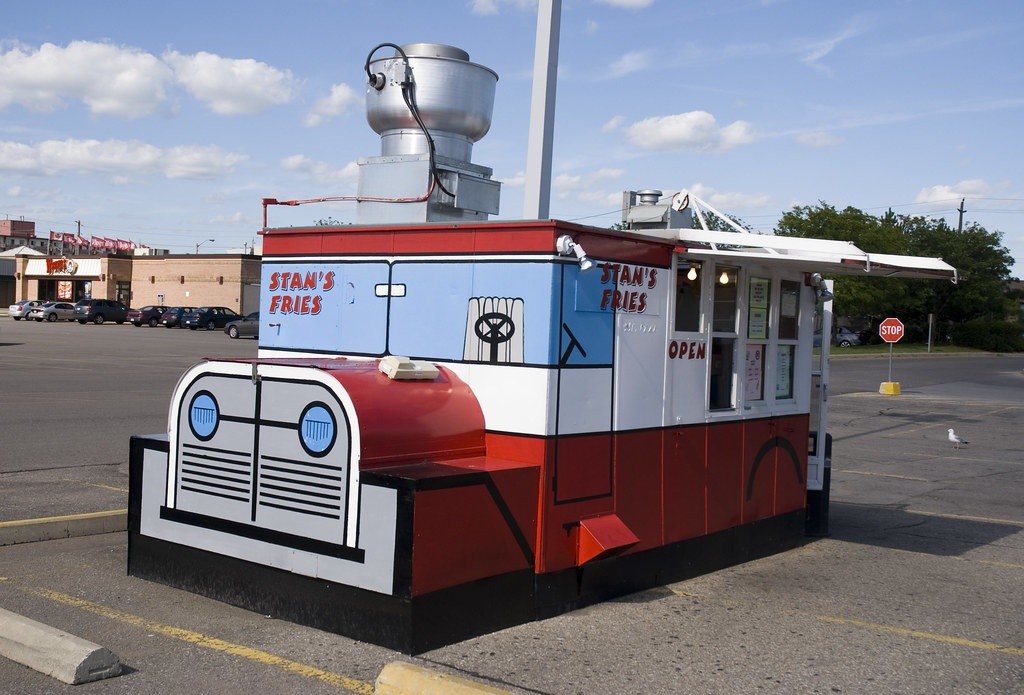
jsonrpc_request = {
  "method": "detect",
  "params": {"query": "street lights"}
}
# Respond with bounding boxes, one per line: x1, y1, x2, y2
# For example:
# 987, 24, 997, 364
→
196, 239, 215, 254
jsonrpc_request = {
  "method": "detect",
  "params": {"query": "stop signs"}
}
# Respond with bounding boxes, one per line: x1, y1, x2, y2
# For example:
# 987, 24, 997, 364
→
878, 317, 904, 343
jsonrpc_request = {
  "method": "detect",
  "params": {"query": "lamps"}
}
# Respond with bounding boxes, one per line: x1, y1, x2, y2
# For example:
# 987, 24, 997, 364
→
557, 236, 598, 274
808, 270, 834, 302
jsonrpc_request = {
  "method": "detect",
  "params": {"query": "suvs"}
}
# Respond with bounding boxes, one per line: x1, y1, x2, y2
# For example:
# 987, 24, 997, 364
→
73, 299, 135, 325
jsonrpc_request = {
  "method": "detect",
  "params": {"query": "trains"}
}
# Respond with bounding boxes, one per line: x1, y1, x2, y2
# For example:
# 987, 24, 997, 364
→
127, 39, 955, 603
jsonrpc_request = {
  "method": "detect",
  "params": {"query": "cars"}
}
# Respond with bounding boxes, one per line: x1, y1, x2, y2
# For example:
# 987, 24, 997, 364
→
28, 301, 77, 322
127, 306, 172, 327
812, 326, 872, 347
182, 307, 247, 331
159, 307, 199, 329
9, 300, 49, 321
224, 312, 259, 340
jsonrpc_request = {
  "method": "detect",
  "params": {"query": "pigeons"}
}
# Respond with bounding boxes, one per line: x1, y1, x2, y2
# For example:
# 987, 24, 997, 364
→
947, 429, 971, 450
